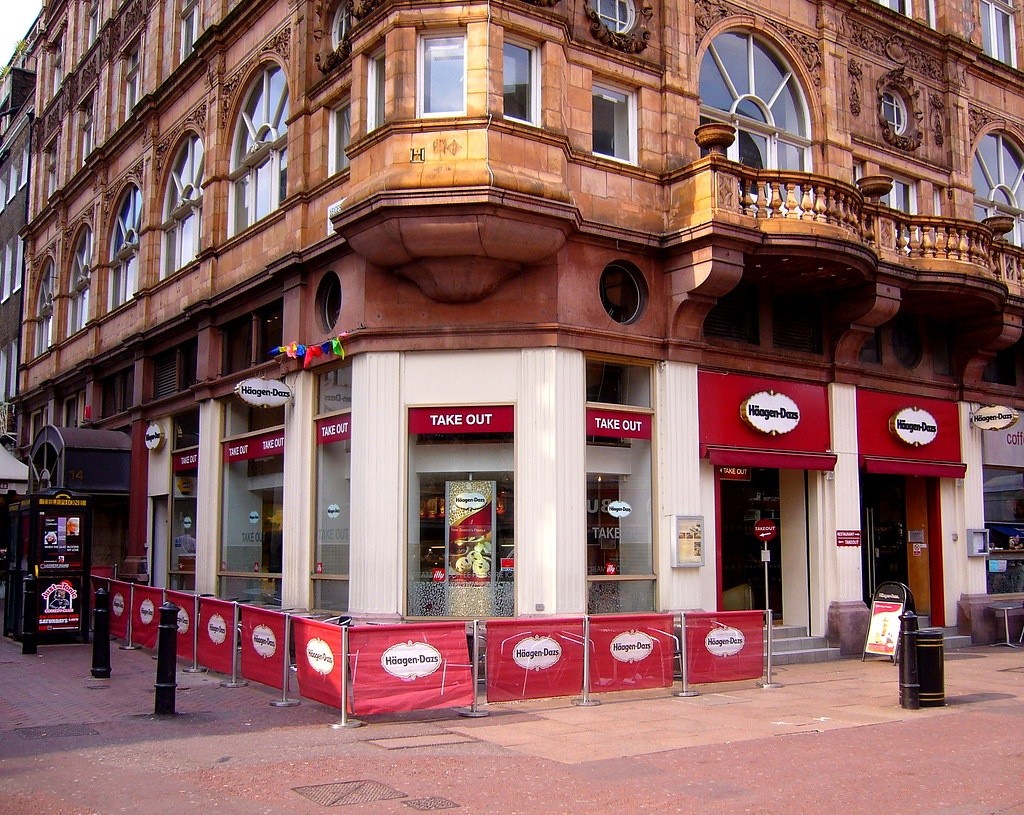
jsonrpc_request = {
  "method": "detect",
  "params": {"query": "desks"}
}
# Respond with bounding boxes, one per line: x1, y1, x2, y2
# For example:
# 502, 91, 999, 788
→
988, 602, 1023, 649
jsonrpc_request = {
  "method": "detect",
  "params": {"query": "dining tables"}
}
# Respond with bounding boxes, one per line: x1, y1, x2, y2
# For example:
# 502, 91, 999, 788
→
599, 629, 634, 686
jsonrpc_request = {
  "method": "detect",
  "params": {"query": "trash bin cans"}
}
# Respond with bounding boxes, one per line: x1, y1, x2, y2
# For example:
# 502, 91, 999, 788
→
916, 629, 946, 707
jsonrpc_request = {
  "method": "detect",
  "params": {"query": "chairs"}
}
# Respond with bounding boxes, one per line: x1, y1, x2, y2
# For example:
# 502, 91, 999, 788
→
441, 633, 488, 697
553, 631, 600, 692
491, 630, 552, 695
180, 590, 382, 668
632, 627, 682, 686
